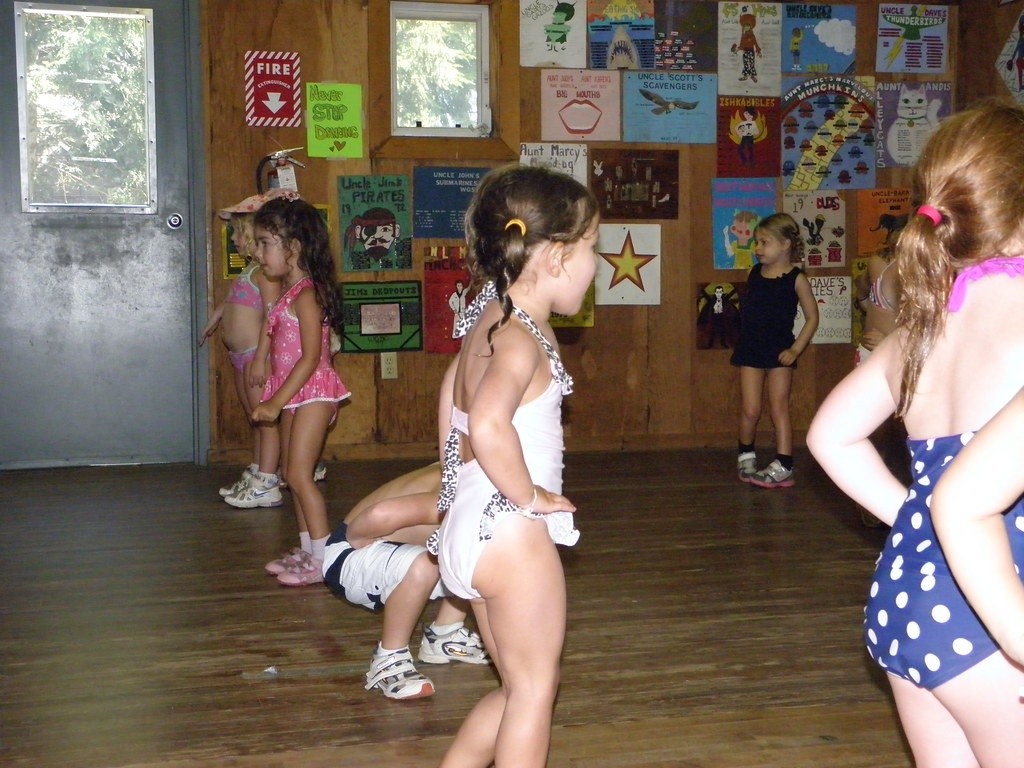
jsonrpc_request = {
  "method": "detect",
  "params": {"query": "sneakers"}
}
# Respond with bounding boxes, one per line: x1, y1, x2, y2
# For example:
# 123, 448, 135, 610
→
224, 472, 284, 508
736, 451, 758, 483
750, 459, 796, 487
276, 556, 324, 585
265, 548, 313, 575
218, 465, 257, 497
418, 626, 494, 663
276, 459, 328, 488
364, 641, 435, 701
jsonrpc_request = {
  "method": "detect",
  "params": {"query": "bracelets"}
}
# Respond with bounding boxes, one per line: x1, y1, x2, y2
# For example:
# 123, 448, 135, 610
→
532, 488, 537, 506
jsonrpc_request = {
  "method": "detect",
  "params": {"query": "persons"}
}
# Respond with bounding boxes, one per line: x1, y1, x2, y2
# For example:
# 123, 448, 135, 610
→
427, 165, 599, 768
806, 101, 1024, 768
203, 190, 351, 584
322, 461, 498, 702
730, 212, 819, 487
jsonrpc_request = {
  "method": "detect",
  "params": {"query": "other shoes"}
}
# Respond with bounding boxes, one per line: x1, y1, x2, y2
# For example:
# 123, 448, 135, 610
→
857, 503, 883, 527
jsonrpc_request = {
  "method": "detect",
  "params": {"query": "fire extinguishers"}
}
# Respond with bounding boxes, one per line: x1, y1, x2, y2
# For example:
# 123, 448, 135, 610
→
256, 146, 306, 195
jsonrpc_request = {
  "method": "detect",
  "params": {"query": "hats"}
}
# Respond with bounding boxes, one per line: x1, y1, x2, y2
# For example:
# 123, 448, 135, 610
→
219, 193, 273, 220
